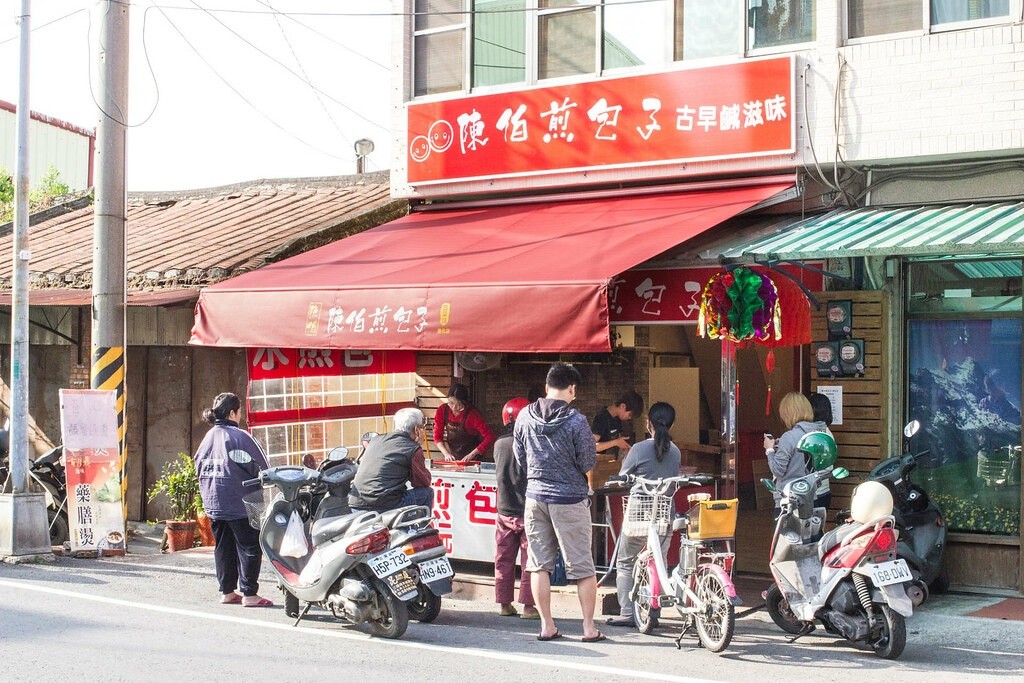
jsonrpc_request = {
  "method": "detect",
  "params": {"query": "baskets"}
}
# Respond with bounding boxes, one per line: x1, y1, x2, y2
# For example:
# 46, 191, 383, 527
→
242, 487, 282, 530
622, 494, 670, 537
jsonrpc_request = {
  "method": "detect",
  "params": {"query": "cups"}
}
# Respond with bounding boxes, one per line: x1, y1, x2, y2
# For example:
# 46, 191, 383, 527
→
107, 531, 124, 549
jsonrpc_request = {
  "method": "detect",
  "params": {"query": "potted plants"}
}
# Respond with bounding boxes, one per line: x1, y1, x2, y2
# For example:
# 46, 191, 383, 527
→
146, 452, 218, 553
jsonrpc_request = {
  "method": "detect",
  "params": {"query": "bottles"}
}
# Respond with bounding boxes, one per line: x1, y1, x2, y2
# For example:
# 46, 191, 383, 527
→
760, 478, 776, 491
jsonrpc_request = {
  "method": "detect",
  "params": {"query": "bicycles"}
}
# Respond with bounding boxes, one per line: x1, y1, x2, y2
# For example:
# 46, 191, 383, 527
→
607, 471, 739, 655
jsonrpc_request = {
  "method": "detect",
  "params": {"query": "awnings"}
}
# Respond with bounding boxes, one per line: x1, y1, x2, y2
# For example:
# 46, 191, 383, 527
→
189, 183, 795, 353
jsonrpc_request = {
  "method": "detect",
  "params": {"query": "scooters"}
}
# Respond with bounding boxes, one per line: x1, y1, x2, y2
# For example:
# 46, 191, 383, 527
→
831, 417, 949, 611
227, 430, 456, 640
758, 462, 925, 661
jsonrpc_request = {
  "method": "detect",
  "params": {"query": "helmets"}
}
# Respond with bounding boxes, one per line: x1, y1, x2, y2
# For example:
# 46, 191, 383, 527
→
798, 432, 838, 470
502, 398, 530, 425
849, 480, 895, 522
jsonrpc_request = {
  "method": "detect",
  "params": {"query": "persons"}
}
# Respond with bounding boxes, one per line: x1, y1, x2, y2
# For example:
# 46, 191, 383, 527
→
433, 383, 496, 463
808, 393, 835, 543
348, 408, 435, 514
494, 398, 541, 620
761, 392, 827, 601
194, 393, 274, 607
591, 391, 644, 454
605, 403, 681, 628
512, 362, 607, 642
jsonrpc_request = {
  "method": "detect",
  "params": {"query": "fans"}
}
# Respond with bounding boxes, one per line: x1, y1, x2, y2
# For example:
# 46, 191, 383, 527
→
454, 351, 504, 378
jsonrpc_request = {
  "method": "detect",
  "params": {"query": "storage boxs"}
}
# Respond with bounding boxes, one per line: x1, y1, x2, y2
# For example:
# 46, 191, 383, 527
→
684, 498, 739, 539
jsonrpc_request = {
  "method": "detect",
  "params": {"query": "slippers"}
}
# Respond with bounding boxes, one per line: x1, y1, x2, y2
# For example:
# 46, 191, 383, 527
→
243, 597, 274, 607
220, 594, 243, 603
582, 630, 607, 643
519, 608, 541, 619
500, 606, 518, 616
536, 628, 562, 640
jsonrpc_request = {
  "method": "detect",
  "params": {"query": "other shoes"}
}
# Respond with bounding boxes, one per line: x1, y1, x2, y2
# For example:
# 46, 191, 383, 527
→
642, 617, 661, 629
608, 616, 633, 626
761, 589, 768, 600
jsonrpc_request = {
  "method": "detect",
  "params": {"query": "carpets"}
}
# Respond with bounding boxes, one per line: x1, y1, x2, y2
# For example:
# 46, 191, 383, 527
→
966, 598, 1024, 621
732, 578, 776, 610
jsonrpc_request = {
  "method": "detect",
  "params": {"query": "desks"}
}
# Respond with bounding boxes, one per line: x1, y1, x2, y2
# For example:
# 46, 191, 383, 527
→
590, 473, 718, 589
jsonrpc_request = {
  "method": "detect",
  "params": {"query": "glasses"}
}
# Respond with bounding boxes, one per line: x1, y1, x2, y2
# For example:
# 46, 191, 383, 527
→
572, 388, 577, 401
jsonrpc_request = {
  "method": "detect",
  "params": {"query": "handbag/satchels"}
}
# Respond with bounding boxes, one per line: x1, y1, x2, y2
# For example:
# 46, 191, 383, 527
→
280, 510, 308, 558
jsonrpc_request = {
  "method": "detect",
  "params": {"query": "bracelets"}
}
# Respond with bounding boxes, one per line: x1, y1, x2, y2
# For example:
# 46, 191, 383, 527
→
765, 448, 775, 455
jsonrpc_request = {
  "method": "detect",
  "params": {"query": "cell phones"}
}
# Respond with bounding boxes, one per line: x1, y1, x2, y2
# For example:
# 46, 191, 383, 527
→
766, 433, 780, 447
